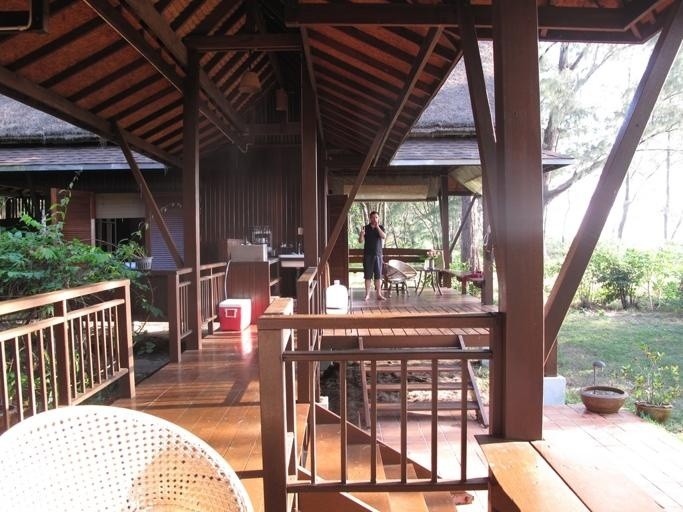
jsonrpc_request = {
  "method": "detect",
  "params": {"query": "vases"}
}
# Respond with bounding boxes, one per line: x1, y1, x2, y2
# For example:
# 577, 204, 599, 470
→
579, 385, 625, 413
428, 258, 433, 270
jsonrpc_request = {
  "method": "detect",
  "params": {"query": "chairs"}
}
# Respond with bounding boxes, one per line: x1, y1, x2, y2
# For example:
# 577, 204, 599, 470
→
1, 404, 258, 512
380, 259, 418, 299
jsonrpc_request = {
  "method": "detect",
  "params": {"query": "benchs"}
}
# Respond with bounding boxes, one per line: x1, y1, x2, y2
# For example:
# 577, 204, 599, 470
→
442, 267, 482, 295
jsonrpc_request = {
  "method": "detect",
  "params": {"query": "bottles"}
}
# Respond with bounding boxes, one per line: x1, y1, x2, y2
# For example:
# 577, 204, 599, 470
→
280, 240, 286, 248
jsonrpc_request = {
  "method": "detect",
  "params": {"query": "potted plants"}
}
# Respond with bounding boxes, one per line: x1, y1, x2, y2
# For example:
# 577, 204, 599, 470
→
134, 246, 153, 270
619, 347, 680, 425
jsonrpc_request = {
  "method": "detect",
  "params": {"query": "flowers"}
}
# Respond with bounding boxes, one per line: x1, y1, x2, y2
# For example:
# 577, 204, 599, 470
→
424, 249, 439, 259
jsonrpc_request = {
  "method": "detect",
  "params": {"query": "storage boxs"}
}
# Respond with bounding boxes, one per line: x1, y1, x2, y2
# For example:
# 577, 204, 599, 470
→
217, 298, 252, 331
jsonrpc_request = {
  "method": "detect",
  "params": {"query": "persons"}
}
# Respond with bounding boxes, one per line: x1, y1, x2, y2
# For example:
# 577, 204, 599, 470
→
357, 211, 387, 301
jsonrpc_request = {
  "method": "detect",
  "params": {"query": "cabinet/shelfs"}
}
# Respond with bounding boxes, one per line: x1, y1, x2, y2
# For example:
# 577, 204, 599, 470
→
227, 257, 281, 326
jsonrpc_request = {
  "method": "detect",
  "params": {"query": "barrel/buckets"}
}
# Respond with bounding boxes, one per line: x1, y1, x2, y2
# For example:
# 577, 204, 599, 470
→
325, 280, 348, 314
